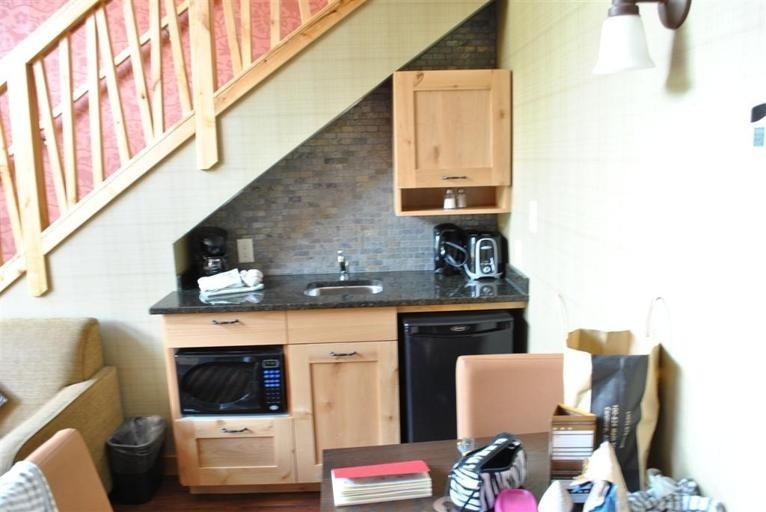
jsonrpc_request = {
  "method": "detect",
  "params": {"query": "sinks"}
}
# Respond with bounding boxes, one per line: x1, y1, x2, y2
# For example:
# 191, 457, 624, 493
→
304, 280, 384, 298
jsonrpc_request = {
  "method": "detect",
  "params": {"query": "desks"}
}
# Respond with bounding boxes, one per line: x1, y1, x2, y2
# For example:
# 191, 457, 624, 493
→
319, 431, 550, 512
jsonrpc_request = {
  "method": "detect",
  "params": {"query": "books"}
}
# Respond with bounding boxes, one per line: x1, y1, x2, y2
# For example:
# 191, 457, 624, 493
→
328, 457, 437, 507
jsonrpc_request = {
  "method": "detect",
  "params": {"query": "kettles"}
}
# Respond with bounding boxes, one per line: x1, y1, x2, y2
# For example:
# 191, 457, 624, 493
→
431, 223, 464, 275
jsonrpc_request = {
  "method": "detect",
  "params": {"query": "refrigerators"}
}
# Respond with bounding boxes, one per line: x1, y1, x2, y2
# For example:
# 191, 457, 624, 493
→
397, 312, 515, 443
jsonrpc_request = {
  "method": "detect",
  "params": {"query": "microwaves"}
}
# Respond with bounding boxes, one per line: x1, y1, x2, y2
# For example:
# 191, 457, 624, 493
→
172, 348, 289, 415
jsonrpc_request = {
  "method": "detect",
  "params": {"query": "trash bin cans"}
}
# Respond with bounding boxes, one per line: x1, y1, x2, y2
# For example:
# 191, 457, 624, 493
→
106, 416, 168, 506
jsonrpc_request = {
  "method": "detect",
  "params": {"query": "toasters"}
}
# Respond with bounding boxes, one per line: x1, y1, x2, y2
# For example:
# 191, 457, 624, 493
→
464, 229, 506, 281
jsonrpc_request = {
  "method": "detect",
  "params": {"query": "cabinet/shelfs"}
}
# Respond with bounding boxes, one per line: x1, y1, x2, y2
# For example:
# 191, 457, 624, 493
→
391, 67, 512, 216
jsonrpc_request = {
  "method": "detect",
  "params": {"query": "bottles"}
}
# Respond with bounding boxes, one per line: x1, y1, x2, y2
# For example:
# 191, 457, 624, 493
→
443, 189, 456, 209
456, 189, 467, 208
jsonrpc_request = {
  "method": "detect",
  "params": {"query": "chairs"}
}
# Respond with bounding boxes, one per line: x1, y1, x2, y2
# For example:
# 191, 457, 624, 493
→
0, 317, 126, 495
455, 353, 562, 441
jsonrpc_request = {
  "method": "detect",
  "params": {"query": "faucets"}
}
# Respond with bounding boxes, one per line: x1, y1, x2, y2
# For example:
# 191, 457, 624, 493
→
336, 250, 348, 282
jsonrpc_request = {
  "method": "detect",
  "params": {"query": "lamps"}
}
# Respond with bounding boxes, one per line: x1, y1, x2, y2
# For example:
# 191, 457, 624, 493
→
596, 0, 691, 77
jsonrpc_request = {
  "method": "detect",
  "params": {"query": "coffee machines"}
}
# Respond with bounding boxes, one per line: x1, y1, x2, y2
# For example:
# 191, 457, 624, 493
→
188, 226, 229, 289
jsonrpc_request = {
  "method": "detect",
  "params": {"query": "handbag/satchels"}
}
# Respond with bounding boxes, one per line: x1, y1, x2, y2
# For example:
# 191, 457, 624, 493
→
447, 432, 527, 512
